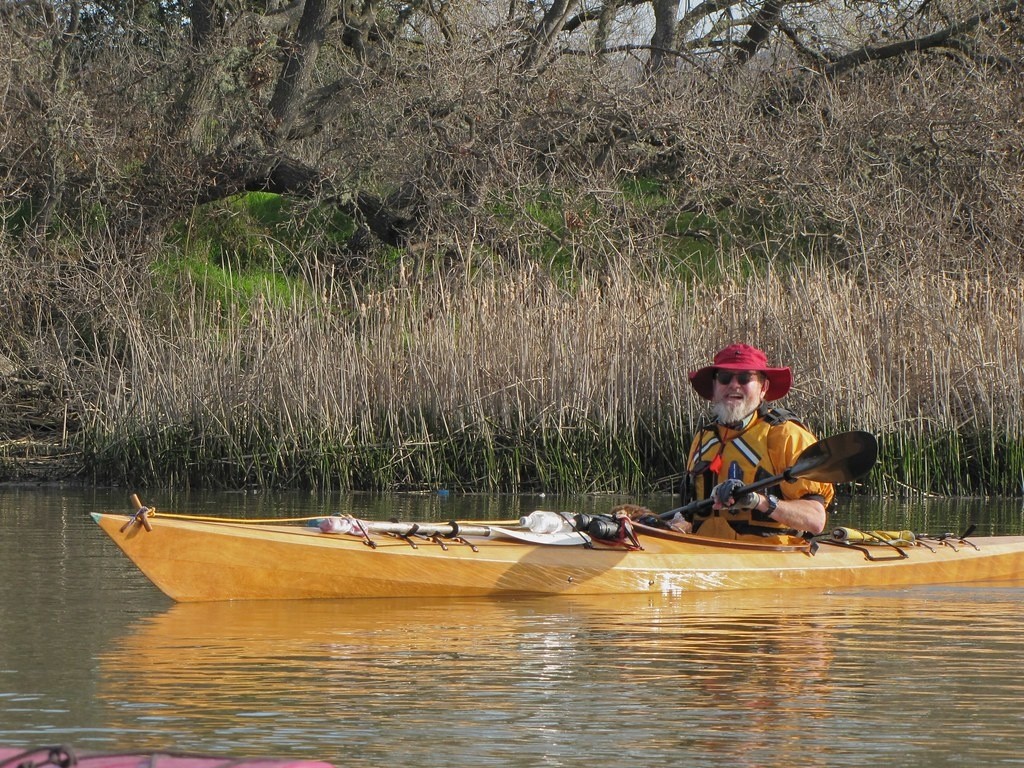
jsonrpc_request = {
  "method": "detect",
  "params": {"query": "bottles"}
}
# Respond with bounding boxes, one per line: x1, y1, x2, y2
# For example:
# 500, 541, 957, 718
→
519, 510, 577, 534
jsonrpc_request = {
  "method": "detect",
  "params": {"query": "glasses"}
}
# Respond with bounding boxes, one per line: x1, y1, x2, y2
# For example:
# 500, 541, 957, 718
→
716, 371, 758, 385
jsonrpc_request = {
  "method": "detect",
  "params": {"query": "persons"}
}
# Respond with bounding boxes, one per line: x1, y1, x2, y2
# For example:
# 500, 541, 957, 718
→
613, 344, 835, 545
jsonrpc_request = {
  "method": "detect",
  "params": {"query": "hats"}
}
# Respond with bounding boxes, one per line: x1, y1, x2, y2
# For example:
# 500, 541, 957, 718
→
687, 343, 792, 401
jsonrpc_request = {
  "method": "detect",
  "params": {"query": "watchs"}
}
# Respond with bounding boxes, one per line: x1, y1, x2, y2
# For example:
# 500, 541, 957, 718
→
762, 494, 779, 515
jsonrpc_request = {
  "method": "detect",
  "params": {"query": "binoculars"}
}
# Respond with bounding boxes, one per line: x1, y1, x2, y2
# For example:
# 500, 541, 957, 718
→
574, 512, 633, 540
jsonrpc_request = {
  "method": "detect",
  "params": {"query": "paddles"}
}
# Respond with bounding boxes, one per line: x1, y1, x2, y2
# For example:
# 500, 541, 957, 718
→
656, 429, 879, 520
319, 516, 592, 546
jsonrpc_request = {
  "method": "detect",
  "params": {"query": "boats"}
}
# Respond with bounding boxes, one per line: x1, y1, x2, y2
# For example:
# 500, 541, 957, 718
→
90, 494, 1024, 603
